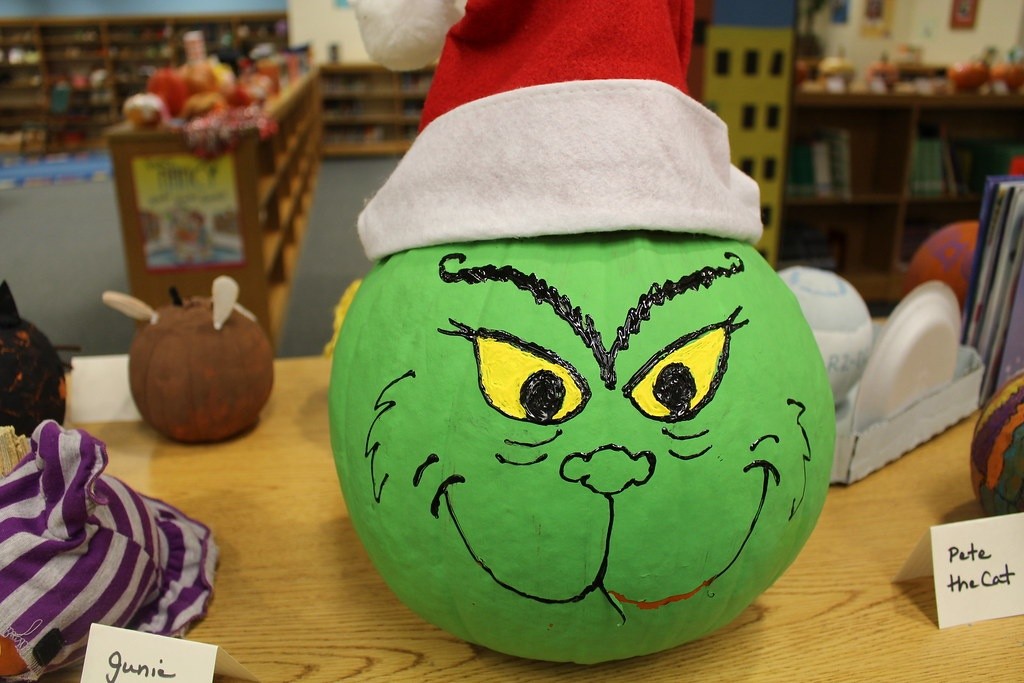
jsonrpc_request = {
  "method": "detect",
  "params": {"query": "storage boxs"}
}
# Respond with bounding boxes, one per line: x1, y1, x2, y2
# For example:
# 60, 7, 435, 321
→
829, 335, 986, 485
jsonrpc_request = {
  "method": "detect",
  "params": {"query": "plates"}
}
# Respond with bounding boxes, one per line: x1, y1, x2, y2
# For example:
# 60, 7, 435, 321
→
848, 279, 962, 431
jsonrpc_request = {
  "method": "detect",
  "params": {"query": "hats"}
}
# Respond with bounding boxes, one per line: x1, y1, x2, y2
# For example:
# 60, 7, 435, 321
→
355, 0, 764, 262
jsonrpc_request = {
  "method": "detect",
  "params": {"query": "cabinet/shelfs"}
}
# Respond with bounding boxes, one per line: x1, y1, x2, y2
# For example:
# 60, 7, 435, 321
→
311, 60, 434, 157
111, 59, 325, 342
1, 11, 293, 160
782, 87, 1014, 299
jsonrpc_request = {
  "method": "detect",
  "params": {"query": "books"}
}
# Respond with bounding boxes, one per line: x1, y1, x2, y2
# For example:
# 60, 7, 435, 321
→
912, 141, 942, 192
786, 130, 852, 199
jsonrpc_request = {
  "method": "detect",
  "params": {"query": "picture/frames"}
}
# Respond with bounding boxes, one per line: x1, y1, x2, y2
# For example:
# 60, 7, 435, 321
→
951, 1, 980, 29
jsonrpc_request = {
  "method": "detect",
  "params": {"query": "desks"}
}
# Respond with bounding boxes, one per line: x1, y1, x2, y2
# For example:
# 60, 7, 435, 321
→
62, 319, 1023, 682
